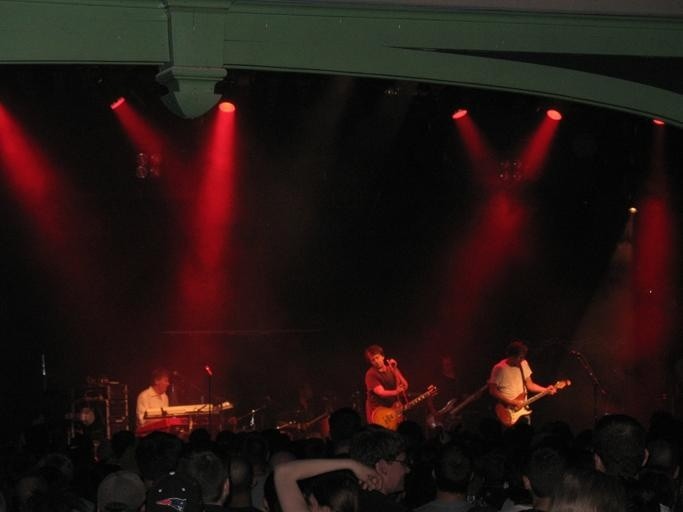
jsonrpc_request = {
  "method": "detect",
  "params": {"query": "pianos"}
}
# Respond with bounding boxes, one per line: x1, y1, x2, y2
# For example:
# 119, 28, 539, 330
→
137, 402, 238, 434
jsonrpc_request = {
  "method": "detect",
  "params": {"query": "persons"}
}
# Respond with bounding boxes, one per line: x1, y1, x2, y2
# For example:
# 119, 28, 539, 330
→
486, 338, 556, 429
274, 385, 333, 441
362, 344, 408, 432
135, 368, 172, 426
427, 354, 485, 420
2, 409, 683, 510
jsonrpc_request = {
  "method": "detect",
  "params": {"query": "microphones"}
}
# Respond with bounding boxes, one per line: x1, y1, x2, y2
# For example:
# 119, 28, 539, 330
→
205, 367, 212, 376
571, 350, 581, 356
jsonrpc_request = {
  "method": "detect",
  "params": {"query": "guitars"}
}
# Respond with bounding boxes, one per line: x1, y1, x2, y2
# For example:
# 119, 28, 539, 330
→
427, 385, 487, 431
496, 380, 571, 427
370, 385, 438, 431
